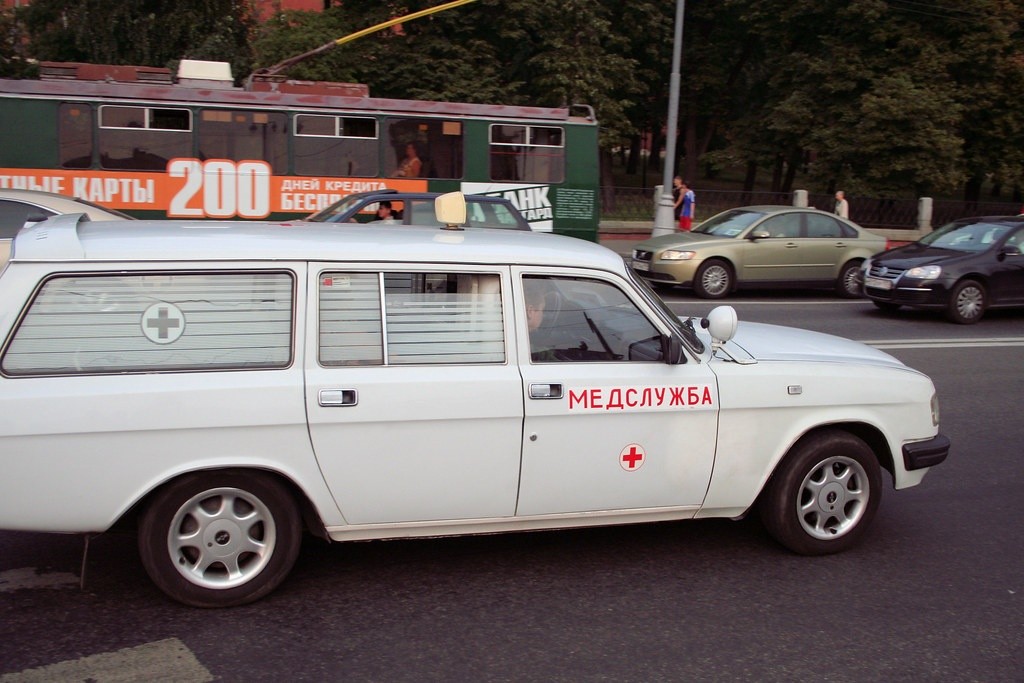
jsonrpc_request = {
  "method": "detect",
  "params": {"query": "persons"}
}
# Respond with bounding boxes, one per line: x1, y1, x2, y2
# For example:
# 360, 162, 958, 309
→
524, 289, 559, 362
391, 141, 422, 177
350, 201, 394, 224
673, 176, 696, 233
834, 191, 849, 219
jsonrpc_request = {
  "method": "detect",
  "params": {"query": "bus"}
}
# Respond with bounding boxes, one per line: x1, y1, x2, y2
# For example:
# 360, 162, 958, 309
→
0, 59, 600, 245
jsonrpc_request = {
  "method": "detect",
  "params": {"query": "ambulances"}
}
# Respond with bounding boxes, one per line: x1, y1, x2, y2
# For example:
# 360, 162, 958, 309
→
1, 212, 953, 610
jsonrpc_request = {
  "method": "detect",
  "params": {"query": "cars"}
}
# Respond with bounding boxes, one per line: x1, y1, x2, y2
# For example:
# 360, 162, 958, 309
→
853, 215, 1024, 325
632, 204, 892, 299
0, 188, 142, 274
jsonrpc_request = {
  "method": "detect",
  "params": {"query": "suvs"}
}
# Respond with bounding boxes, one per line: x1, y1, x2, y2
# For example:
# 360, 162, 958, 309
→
303, 187, 534, 232
